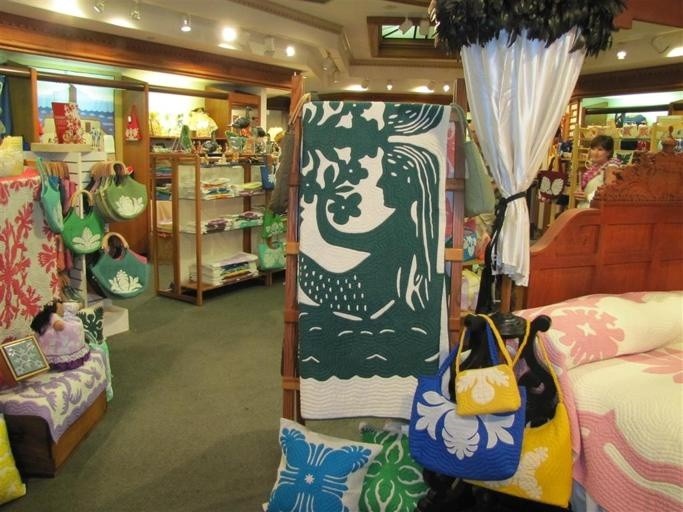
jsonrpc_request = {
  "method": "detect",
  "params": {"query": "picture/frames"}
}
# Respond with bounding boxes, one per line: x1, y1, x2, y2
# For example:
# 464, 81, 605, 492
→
1, 336, 50, 382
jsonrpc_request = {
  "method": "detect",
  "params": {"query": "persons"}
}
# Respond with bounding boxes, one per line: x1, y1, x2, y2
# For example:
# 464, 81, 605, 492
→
574, 134, 621, 209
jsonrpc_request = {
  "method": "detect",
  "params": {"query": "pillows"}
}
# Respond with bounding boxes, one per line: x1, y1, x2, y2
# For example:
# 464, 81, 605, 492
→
259, 414, 383, 512
354, 417, 426, 511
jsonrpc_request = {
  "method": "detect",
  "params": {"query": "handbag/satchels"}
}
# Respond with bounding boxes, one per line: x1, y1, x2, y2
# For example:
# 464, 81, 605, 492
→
259, 155, 279, 191
407, 313, 527, 482
453, 313, 523, 418
33, 156, 66, 235
88, 159, 150, 224
538, 155, 567, 200
87, 232, 149, 301
60, 189, 106, 256
461, 323, 574, 509
260, 200, 288, 239
257, 240, 288, 272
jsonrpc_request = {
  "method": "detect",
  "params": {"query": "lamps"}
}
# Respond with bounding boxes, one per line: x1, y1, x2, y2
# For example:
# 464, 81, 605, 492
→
120, 84, 233, 255
321, 56, 452, 93
398, 11, 430, 35
94, 1, 296, 58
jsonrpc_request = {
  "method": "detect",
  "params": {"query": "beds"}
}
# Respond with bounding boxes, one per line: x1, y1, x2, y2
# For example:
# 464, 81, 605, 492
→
497, 127, 682, 512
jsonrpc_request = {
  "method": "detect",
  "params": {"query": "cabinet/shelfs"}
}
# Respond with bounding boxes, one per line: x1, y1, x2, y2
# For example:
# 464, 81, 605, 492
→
557, 118, 682, 218
149, 151, 273, 305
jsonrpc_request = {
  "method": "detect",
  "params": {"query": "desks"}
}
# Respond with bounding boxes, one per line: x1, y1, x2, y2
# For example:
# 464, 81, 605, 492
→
0, 348, 107, 479
